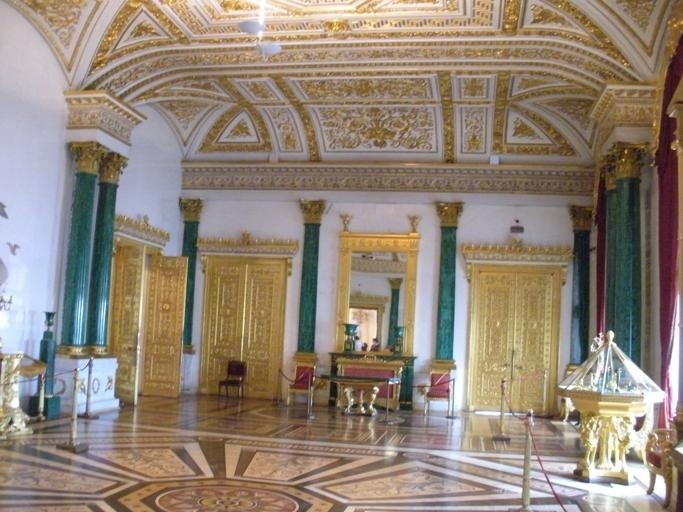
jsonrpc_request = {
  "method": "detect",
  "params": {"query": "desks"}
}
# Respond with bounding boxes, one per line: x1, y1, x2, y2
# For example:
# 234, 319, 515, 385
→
320, 376, 400, 416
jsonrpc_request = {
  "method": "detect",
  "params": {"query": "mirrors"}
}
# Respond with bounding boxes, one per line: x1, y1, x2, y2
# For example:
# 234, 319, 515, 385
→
338, 231, 421, 356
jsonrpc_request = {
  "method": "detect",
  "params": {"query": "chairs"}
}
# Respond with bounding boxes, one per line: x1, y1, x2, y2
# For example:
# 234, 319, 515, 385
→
219, 359, 247, 400
286, 362, 317, 410
425, 371, 451, 418
